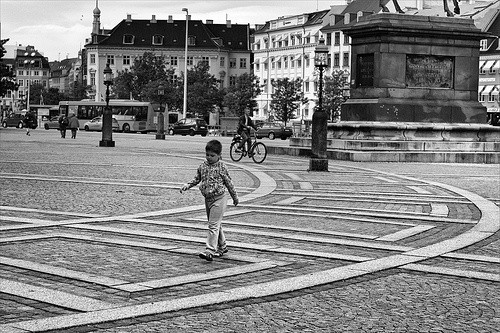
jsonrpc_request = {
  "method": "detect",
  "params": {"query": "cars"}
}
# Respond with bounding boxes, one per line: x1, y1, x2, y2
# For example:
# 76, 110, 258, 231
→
44, 116, 71, 130
84, 116, 119, 133
256, 122, 294, 141
168, 117, 208, 137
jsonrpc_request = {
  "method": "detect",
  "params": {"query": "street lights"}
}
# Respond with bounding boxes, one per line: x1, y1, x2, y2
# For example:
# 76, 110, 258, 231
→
24, 51, 36, 111
98, 61, 116, 147
181, 8, 190, 118
155, 77, 166, 139
310, 34, 330, 171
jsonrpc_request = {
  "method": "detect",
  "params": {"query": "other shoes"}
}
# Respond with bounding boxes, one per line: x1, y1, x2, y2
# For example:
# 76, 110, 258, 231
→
248, 152, 256, 158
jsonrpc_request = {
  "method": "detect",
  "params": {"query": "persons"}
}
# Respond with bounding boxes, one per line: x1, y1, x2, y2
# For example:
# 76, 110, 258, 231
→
24, 111, 34, 136
179, 139, 239, 262
69, 113, 79, 139
59, 114, 68, 138
237, 107, 257, 156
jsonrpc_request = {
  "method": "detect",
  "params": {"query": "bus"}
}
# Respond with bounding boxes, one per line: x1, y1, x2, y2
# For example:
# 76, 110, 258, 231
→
58, 99, 169, 134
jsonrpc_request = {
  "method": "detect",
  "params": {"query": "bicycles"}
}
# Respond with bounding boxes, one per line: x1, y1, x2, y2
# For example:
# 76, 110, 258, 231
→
230, 128, 268, 163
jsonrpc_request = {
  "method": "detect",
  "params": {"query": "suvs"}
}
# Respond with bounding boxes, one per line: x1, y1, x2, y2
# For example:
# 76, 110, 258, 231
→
2, 111, 37, 129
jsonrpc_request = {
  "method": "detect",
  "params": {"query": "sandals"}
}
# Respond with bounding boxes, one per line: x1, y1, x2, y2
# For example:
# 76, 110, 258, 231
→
213, 247, 228, 257
199, 250, 215, 262
